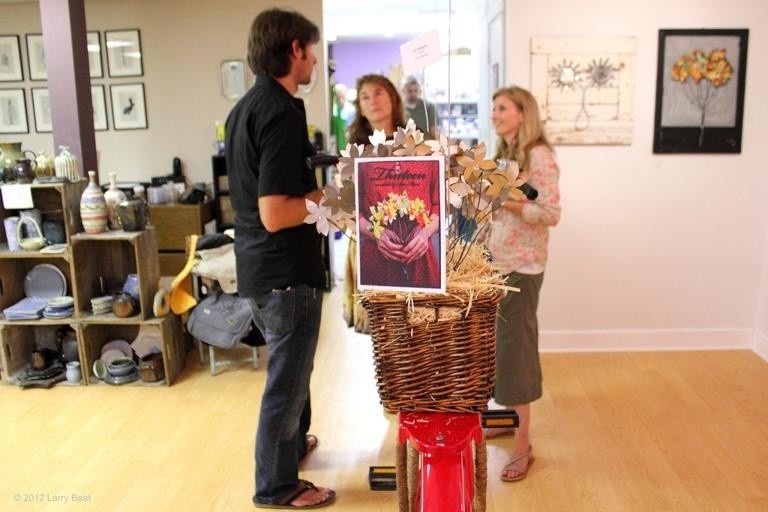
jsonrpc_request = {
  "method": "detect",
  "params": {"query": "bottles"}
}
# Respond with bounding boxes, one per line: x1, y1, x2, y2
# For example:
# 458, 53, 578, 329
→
3, 216, 23, 253
2, 151, 54, 184
65, 361, 82, 383
122, 272, 140, 304
43, 218, 65, 244
79, 169, 145, 233
54, 327, 79, 367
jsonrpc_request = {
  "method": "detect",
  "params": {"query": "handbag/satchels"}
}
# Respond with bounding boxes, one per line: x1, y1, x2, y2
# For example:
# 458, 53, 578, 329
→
187, 293, 253, 350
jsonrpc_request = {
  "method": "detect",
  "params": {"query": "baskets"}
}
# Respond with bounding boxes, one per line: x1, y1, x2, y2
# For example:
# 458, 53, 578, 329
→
359, 287, 506, 413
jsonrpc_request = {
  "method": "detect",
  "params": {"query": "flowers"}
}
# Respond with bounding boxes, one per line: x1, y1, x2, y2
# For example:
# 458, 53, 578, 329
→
303, 117, 527, 271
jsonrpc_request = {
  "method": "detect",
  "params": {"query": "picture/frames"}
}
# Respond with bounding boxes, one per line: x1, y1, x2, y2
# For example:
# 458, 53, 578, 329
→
0, 33, 53, 134
86, 28, 148, 131
528, 34, 635, 145
653, 28, 749, 155
353, 155, 447, 295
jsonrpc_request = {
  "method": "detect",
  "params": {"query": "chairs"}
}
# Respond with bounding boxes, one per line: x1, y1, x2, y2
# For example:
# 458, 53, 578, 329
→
191, 275, 260, 377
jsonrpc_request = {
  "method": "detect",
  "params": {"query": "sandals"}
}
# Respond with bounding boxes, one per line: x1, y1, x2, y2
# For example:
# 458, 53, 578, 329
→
500, 445, 534, 481
485, 428, 514, 438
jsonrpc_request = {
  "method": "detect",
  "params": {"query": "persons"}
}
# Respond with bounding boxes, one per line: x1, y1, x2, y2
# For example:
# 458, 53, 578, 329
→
224, 7, 337, 510
400, 75, 438, 140
341, 75, 435, 334
359, 160, 440, 290
470, 85, 560, 482
334, 83, 357, 154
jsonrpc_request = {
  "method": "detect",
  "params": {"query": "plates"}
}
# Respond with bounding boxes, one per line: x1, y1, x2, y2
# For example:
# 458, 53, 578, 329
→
43, 296, 74, 319
99, 339, 133, 365
41, 367, 64, 379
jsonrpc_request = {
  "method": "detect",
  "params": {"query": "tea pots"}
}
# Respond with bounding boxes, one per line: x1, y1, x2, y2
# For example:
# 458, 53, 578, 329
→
113, 291, 139, 317
0, 140, 38, 171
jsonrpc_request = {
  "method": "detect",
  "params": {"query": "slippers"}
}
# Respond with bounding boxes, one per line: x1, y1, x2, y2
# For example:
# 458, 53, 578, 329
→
253, 479, 335, 509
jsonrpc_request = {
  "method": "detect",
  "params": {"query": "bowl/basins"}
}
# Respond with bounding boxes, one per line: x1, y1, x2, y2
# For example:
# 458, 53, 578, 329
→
93, 351, 164, 385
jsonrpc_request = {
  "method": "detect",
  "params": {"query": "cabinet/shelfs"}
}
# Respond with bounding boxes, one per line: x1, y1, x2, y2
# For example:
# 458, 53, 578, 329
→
0, 171, 215, 386
212, 154, 332, 292
